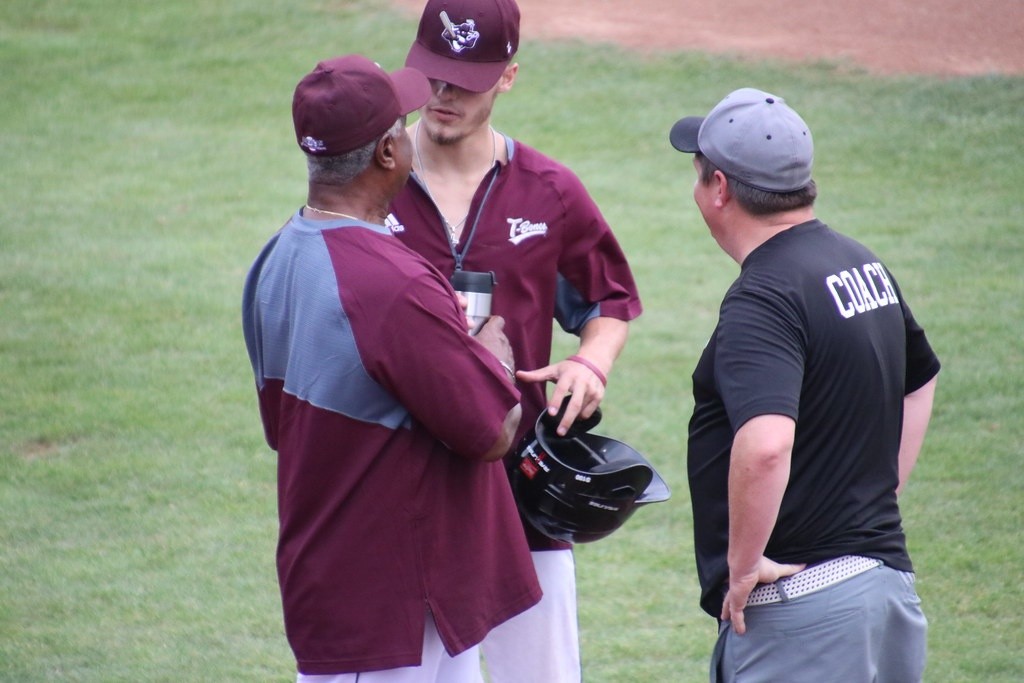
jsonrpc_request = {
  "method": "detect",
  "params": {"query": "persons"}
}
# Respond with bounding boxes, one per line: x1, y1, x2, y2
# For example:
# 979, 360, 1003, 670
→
670, 87, 941, 683
241, 55, 543, 683
404, 0, 643, 683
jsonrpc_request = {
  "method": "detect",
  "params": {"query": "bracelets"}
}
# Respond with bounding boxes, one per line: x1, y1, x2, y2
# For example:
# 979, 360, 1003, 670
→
500, 361, 515, 380
567, 355, 607, 387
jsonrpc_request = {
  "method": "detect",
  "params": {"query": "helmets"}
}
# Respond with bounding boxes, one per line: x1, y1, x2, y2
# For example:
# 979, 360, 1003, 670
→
508, 395, 671, 545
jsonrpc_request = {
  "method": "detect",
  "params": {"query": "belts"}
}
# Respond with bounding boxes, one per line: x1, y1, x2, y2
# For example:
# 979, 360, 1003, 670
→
746, 554, 883, 606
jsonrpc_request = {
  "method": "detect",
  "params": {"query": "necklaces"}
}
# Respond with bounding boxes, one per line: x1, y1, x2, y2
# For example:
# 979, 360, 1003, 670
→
307, 205, 359, 220
415, 118, 497, 246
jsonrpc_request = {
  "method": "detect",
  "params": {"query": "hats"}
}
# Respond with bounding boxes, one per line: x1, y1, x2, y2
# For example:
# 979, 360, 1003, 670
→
670, 87, 815, 192
405, 0, 520, 93
292, 55, 432, 155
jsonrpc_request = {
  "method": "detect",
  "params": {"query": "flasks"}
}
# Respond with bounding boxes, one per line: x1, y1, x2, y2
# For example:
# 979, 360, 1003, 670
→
451, 271, 497, 337
